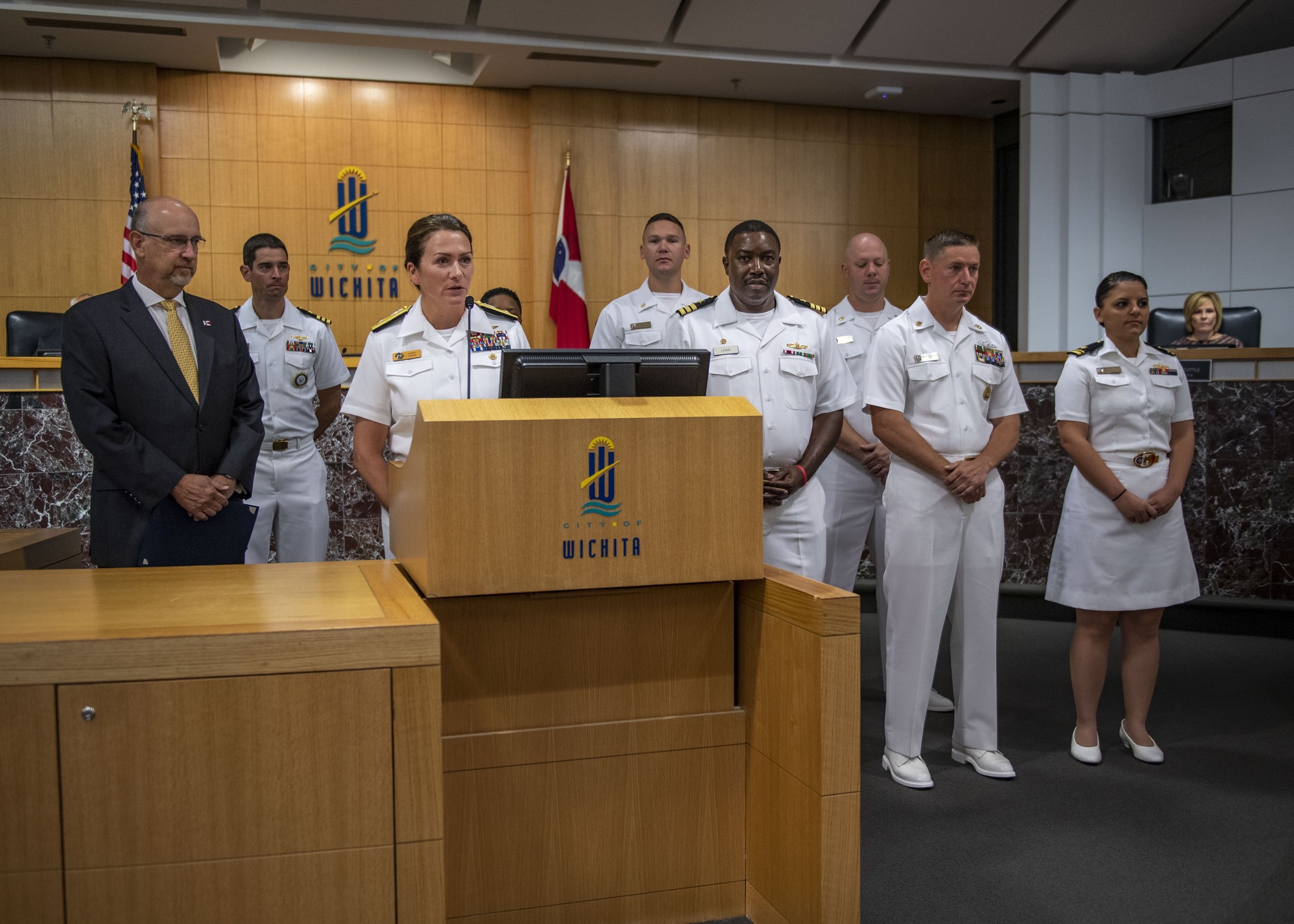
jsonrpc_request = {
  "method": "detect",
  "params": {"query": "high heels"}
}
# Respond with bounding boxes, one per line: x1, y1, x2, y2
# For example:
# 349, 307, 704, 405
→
1071, 726, 1102, 763
1119, 719, 1164, 763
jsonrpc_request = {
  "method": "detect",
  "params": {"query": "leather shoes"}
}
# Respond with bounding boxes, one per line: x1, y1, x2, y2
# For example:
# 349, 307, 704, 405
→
882, 745, 933, 788
886, 687, 954, 712
951, 738, 1015, 778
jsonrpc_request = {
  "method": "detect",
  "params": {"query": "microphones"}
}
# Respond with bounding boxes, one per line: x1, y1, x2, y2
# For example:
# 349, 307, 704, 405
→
464, 296, 474, 399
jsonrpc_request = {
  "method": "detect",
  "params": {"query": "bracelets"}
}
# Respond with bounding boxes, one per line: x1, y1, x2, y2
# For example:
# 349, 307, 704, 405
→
1112, 489, 1126, 502
795, 465, 807, 487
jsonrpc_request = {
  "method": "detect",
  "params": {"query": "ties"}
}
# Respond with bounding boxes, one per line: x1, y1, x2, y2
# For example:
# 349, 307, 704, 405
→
158, 299, 199, 406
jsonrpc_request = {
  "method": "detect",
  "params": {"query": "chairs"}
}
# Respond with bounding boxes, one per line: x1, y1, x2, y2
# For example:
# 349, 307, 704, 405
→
1147, 307, 1261, 348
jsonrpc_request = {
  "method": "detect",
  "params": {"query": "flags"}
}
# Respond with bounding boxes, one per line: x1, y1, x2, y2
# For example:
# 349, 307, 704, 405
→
120, 144, 148, 285
548, 167, 591, 350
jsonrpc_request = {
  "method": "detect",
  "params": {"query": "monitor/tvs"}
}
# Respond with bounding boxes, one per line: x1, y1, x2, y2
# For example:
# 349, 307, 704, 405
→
500, 349, 711, 397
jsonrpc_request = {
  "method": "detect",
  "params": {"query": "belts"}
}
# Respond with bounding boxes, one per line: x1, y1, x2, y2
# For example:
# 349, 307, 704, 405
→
262, 434, 316, 450
1099, 451, 1169, 467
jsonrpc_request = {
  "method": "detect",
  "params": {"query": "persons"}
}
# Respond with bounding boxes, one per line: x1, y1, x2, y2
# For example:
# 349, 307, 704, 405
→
1046, 270, 1201, 763
1170, 291, 1244, 349
339, 214, 533, 560
663, 219, 861, 582
861, 229, 1030, 788
60, 196, 266, 569
480, 287, 522, 326
589, 213, 712, 349
821, 232, 958, 713
226, 234, 350, 564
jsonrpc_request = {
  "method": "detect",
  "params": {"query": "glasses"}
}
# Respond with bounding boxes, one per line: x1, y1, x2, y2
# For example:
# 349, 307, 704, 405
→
141, 234, 207, 249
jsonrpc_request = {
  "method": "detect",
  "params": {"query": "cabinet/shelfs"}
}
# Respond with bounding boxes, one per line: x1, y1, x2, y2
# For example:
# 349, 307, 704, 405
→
1, 559, 442, 924
